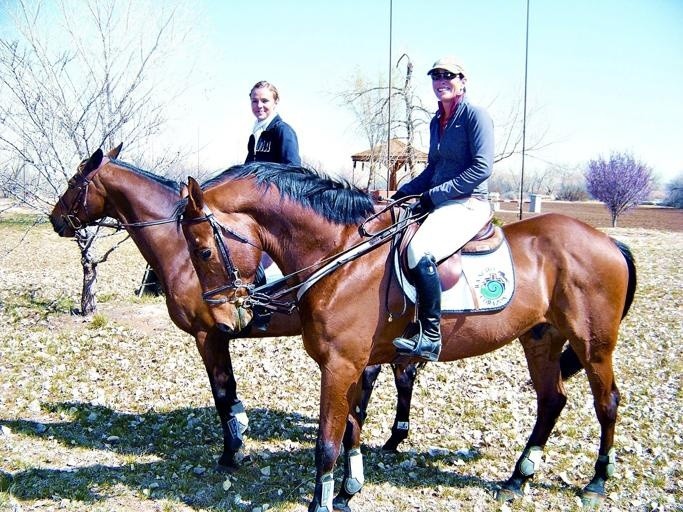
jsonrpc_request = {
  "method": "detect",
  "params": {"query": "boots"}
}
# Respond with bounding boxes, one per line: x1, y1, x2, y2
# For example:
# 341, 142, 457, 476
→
392, 254, 442, 361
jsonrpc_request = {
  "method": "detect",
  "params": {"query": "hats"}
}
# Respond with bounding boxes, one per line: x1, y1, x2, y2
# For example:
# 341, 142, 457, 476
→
427, 55, 465, 76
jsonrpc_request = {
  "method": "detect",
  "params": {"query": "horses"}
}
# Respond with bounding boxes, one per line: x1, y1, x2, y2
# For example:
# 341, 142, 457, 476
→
175, 159, 639, 512
45, 141, 419, 473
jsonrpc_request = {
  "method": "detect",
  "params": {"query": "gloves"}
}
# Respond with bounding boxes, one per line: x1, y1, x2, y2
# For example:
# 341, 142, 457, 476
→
420, 191, 435, 211
391, 191, 407, 204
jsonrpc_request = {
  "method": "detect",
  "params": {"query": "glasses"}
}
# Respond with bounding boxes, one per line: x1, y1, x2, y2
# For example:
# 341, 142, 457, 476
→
431, 71, 458, 81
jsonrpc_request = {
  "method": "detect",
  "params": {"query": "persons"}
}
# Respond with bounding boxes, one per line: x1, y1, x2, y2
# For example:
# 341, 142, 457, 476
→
246, 81, 301, 331
391, 58, 496, 361
